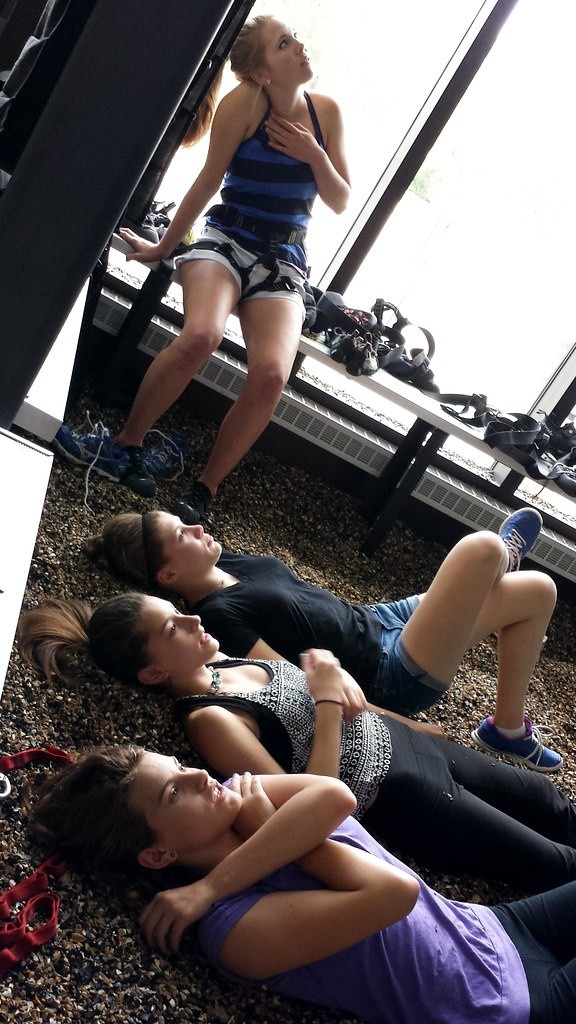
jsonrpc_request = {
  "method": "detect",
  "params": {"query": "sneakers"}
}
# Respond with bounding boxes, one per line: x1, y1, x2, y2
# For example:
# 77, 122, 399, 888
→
497, 507, 543, 572
170, 480, 212, 524
53, 411, 129, 512
110, 435, 155, 495
471, 717, 564, 772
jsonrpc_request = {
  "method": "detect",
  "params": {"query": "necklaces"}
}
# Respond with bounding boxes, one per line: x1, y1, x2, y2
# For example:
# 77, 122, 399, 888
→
217, 566, 225, 590
205, 666, 221, 696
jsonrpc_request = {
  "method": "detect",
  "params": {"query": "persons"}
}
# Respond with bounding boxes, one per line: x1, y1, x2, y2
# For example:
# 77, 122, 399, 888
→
110, 12, 353, 514
23, 505, 576, 1024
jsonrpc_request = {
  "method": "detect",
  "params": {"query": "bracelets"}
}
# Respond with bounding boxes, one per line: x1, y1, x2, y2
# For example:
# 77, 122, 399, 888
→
313, 699, 343, 708
